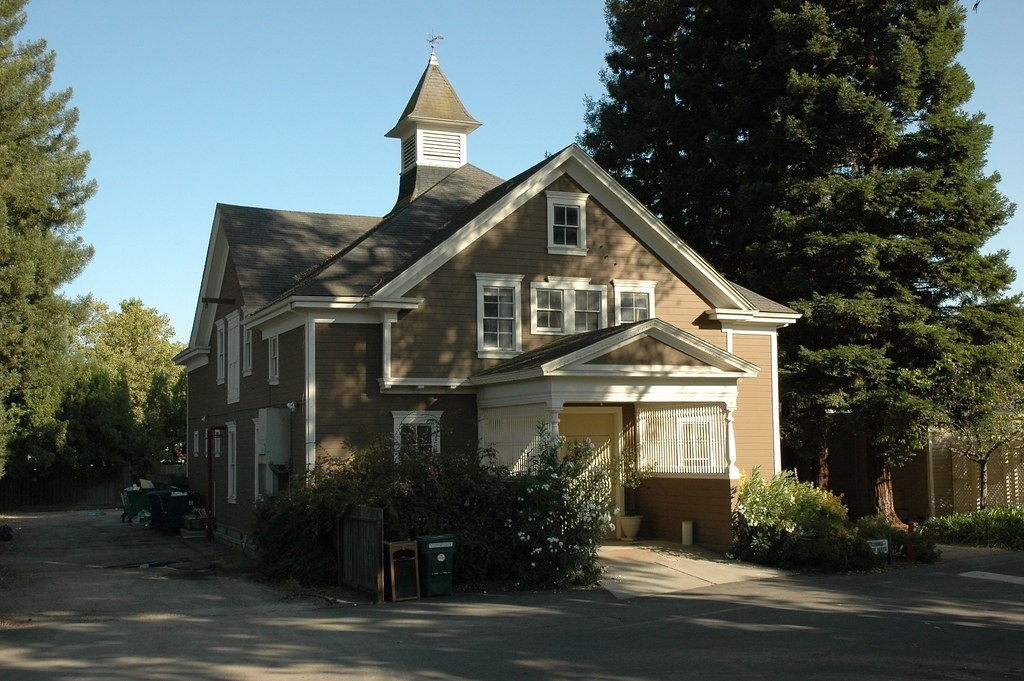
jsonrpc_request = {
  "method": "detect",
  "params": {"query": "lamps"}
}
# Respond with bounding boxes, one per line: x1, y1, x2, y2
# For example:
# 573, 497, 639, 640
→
286, 401, 298, 412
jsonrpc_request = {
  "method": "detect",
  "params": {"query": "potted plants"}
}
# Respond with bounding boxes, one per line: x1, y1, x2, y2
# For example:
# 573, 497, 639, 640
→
602, 447, 658, 542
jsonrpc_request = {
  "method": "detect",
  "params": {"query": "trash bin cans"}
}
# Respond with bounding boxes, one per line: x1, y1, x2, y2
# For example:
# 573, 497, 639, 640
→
417, 534, 456, 596
384, 539, 420, 602
146, 490, 197, 531
120, 490, 147, 522
